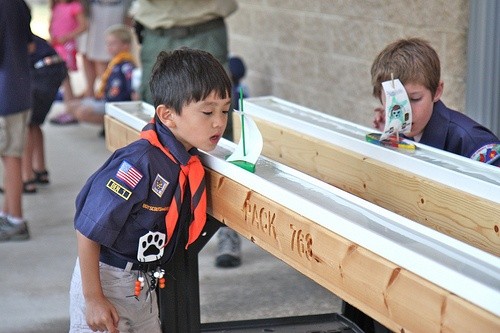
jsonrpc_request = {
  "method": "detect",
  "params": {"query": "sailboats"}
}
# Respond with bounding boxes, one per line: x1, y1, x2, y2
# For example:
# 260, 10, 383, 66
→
222, 85, 264, 171
364, 72, 417, 153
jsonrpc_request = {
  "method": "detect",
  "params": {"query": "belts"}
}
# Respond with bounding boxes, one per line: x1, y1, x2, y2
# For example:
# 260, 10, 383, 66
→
140, 17, 222, 40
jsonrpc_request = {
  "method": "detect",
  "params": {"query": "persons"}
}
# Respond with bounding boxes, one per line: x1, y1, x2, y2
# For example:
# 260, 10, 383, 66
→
68, 46, 233, 333
50, 0, 136, 136
343, 38, 500, 333
127, 0, 248, 269
0, 0, 67, 238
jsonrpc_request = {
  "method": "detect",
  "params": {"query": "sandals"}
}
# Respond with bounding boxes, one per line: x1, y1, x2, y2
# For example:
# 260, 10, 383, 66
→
34, 170, 50, 184
22, 176, 37, 194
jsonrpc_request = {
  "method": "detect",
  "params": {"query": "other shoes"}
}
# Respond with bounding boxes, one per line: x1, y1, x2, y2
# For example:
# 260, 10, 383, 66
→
0, 212, 30, 243
214, 228, 242, 269
51, 111, 78, 125
